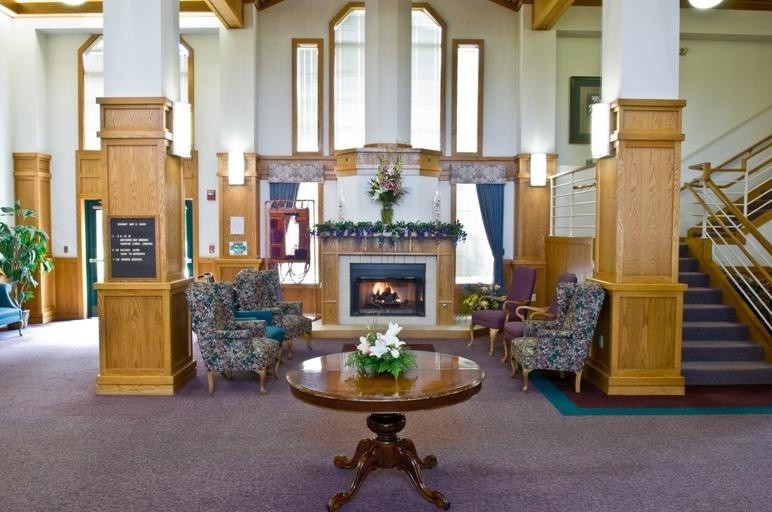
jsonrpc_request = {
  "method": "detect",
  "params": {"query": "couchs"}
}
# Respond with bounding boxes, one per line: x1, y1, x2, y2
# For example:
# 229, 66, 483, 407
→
0, 282, 24, 337
188, 280, 280, 396
231, 309, 284, 344
511, 281, 604, 394
233, 266, 313, 361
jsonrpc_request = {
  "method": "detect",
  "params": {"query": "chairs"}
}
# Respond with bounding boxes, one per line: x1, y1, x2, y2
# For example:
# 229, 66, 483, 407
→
466, 267, 537, 356
502, 273, 577, 362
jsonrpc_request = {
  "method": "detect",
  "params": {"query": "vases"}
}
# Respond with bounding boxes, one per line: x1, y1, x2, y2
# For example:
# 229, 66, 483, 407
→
381, 202, 393, 224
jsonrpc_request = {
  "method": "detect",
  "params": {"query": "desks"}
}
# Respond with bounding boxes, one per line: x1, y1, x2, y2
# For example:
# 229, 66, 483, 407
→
285, 347, 487, 512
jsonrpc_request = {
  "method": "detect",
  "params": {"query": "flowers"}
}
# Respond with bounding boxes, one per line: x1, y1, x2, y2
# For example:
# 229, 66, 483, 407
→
341, 322, 419, 381
367, 141, 409, 203
305, 218, 467, 250
460, 280, 501, 310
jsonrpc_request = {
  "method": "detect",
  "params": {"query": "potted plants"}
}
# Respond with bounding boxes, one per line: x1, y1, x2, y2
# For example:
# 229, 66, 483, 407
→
0, 200, 54, 329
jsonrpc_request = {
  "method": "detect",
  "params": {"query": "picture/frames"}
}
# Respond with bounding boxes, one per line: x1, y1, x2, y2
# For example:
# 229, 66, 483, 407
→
106, 213, 160, 283
569, 74, 603, 144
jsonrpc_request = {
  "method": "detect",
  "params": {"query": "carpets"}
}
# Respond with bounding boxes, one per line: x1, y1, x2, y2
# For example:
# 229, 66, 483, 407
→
528, 366, 772, 417
341, 343, 435, 354
